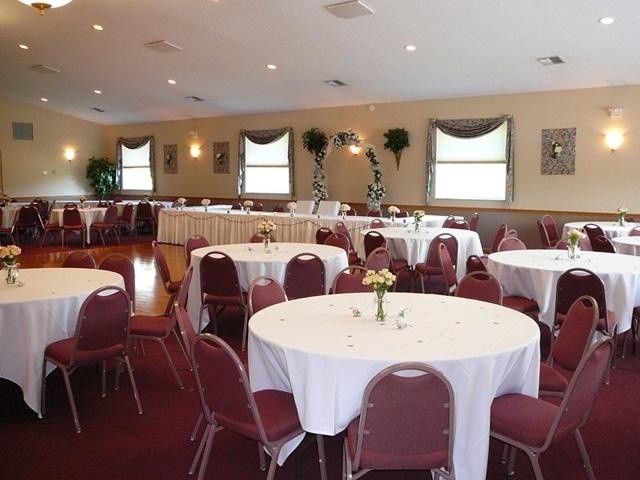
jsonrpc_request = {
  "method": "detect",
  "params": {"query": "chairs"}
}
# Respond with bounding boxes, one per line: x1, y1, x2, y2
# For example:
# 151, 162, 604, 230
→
173, 300, 249, 475
41, 285, 144, 433
342, 362, 455, 479
189, 334, 328, 480
490, 335, 615, 480
500, 295, 599, 464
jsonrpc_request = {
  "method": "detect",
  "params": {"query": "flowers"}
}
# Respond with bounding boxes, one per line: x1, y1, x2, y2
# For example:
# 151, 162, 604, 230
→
312, 130, 386, 210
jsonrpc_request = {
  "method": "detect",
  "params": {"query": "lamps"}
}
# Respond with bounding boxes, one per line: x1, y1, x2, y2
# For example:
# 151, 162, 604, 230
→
18, 0, 72, 16
